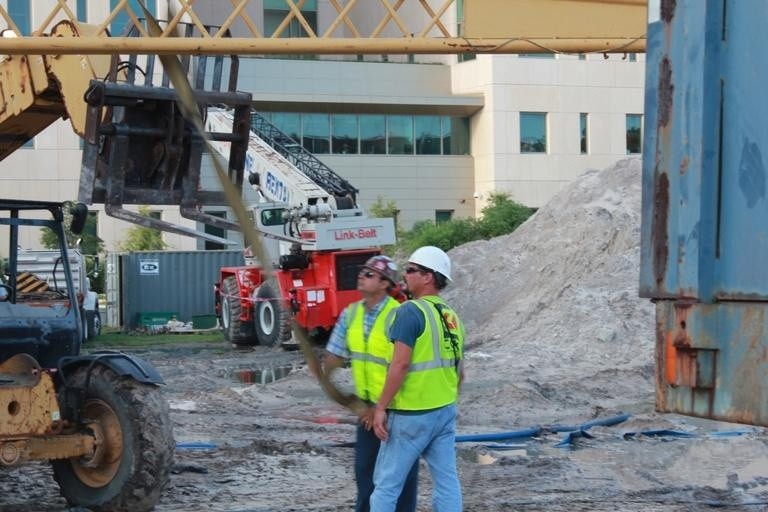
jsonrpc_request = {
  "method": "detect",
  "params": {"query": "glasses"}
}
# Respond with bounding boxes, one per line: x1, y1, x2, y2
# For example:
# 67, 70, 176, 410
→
359, 270, 377, 281
406, 268, 433, 274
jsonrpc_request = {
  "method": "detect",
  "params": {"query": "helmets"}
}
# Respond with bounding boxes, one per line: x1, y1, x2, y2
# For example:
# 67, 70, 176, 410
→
407, 246, 454, 287
356, 254, 402, 288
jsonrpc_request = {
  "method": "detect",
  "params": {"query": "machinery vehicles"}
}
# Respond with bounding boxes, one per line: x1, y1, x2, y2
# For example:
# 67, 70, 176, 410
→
0, 16, 253, 511
203, 89, 415, 347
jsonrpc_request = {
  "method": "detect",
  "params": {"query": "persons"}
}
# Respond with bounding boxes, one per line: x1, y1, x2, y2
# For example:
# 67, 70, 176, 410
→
368, 247, 468, 511
320, 255, 419, 512
